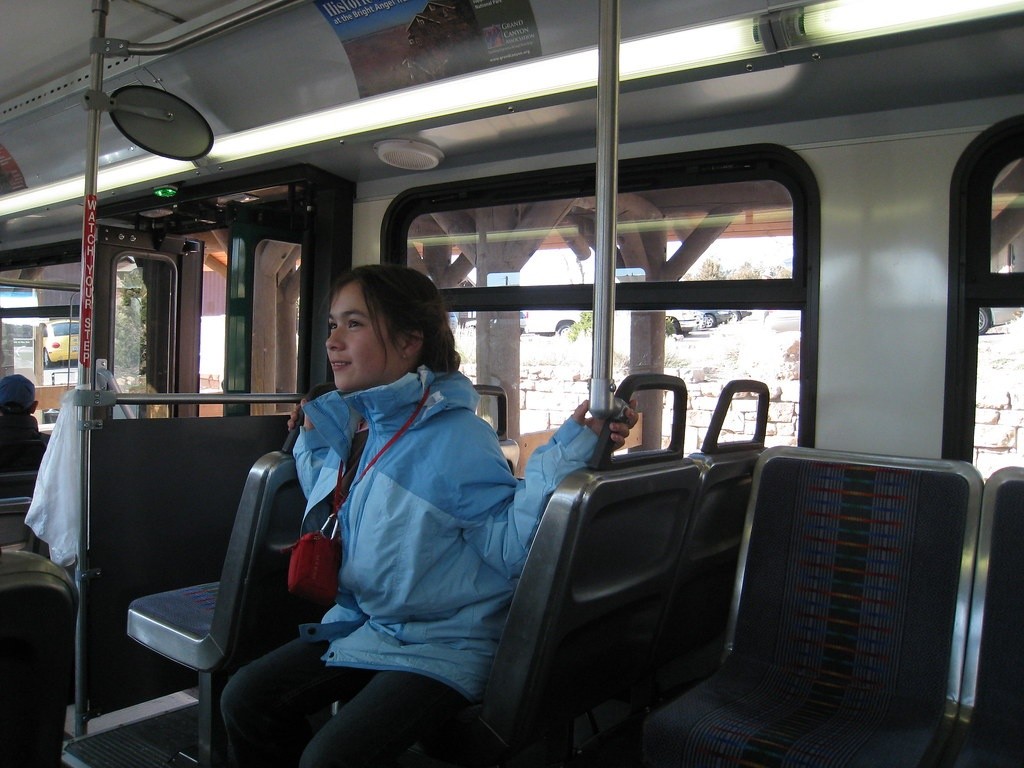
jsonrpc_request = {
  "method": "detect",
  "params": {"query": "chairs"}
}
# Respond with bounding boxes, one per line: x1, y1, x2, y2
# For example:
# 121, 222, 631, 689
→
0, 374, 1024, 768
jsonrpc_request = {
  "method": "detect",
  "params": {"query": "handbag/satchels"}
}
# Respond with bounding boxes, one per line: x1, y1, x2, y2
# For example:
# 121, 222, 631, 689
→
287, 531, 341, 602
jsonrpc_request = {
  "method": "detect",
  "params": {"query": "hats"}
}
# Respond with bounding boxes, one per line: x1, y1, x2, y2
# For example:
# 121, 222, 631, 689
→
0, 374, 36, 412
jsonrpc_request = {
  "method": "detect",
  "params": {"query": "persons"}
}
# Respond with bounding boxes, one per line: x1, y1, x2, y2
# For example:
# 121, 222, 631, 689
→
0, 375, 51, 474
219, 266, 639, 768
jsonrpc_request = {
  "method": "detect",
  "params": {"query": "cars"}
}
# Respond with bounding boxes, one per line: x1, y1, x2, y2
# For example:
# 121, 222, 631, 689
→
445, 276, 752, 338
763, 303, 1024, 336
41, 318, 81, 370
